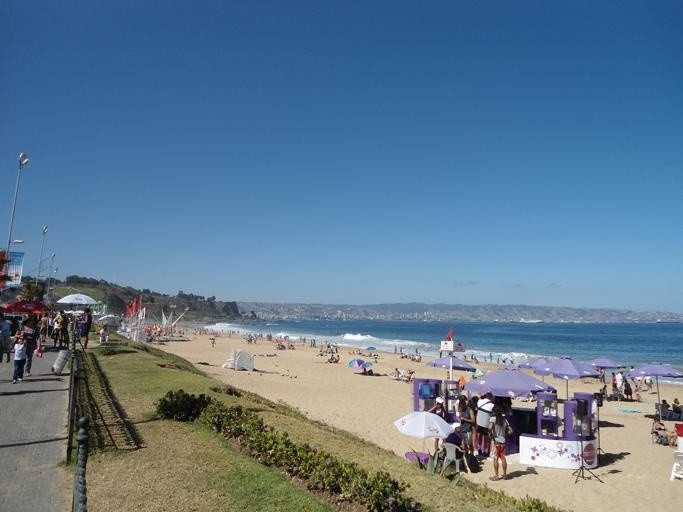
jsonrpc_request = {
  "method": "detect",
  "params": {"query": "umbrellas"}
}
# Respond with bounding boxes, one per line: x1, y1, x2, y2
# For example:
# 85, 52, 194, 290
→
533, 353, 602, 401
627, 360, 683, 404
585, 354, 628, 384
56, 292, 99, 330
393, 408, 456, 457
518, 356, 552, 383
426, 354, 476, 374
462, 366, 557, 403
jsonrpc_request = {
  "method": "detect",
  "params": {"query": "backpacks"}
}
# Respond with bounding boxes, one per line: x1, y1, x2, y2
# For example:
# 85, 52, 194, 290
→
467, 453, 480, 473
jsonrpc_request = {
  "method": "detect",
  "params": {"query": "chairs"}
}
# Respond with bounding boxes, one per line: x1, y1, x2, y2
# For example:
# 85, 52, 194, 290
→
668, 450, 683, 483
655, 403, 683, 421
440, 443, 471, 476
652, 422, 664, 444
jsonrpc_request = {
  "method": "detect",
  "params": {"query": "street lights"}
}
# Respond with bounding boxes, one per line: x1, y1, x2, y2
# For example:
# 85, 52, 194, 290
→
36, 225, 48, 288
46, 253, 58, 301
3, 153, 30, 277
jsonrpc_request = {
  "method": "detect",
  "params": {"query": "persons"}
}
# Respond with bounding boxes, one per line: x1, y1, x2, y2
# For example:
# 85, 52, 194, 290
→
445, 422, 482, 473
427, 396, 447, 450
593, 365, 683, 449
487, 406, 513, 480
455, 391, 494, 457
0, 306, 92, 383
439, 350, 515, 365
195, 328, 422, 384
99, 325, 109, 344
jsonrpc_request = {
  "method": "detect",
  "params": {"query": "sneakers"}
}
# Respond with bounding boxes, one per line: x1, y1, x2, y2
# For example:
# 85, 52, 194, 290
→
13, 373, 32, 384
489, 474, 508, 481
478, 449, 489, 457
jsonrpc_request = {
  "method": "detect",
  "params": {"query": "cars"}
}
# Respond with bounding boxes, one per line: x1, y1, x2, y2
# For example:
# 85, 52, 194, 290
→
99, 315, 119, 320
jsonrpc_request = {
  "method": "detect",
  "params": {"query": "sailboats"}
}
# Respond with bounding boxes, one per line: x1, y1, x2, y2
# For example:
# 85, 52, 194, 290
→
155, 309, 190, 340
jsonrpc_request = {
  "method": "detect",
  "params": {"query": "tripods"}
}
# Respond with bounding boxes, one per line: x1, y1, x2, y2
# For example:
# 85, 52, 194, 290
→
572, 415, 604, 483
597, 406, 614, 463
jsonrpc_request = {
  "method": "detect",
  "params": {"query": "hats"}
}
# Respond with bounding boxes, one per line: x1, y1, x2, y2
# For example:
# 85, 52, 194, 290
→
23, 316, 39, 325
436, 397, 444, 404
494, 437, 506, 444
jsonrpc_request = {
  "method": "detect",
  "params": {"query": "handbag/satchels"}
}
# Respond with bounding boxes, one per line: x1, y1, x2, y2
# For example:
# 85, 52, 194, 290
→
33, 348, 42, 358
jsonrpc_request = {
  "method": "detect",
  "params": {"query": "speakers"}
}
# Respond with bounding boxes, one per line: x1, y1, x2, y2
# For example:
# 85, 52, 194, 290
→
594, 393, 603, 406
577, 399, 588, 415
